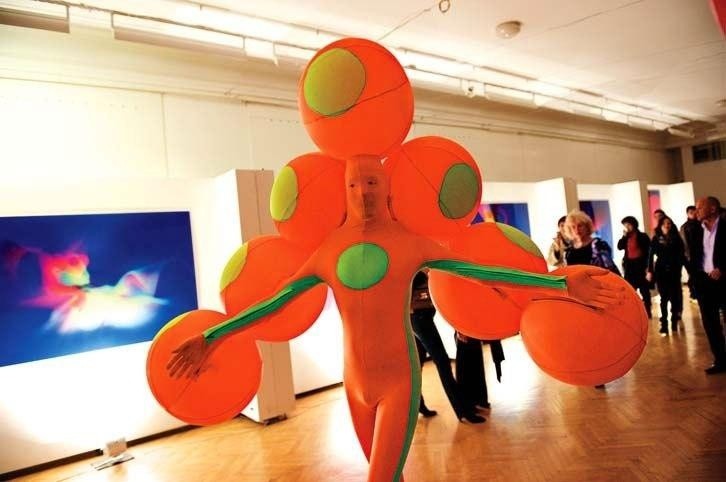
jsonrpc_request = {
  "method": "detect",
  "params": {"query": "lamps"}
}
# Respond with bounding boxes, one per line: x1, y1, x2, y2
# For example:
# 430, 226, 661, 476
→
495, 22, 521, 39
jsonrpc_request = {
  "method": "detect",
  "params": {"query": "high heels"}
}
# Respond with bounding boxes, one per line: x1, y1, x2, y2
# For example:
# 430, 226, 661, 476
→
458, 412, 487, 425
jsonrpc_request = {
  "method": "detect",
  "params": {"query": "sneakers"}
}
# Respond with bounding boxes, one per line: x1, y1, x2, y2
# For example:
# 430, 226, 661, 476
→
704, 364, 725, 375
646, 310, 683, 335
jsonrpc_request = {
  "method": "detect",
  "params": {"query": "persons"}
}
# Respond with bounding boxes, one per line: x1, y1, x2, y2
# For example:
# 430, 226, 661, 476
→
409, 272, 491, 424
548, 196, 726, 388
164, 149, 631, 482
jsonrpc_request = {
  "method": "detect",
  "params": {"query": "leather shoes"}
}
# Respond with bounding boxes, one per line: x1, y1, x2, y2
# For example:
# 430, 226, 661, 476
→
418, 399, 437, 417
